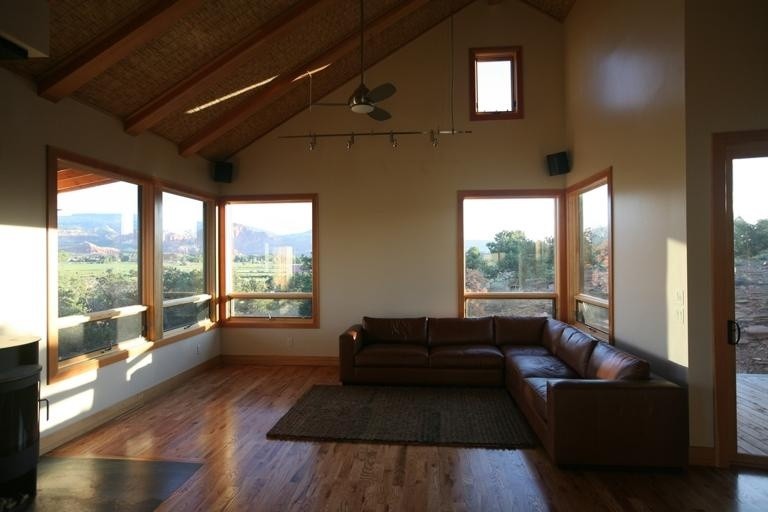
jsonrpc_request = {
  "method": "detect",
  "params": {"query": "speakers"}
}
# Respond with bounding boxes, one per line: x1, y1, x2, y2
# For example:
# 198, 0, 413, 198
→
546, 151, 570, 175
212, 161, 232, 183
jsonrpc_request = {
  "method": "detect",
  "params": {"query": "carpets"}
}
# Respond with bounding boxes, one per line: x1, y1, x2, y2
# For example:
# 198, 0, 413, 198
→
26, 454, 205, 512
265, 381, 536, 449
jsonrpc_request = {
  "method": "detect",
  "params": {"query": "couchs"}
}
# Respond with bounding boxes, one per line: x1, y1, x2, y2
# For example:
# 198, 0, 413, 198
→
341, 315, 691, 477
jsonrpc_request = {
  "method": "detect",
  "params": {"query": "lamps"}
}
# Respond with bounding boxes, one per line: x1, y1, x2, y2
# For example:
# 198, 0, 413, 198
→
275, 127, 469, 151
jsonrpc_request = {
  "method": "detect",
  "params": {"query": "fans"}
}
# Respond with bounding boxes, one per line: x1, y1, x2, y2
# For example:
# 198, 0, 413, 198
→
312, 1, 396, 122
